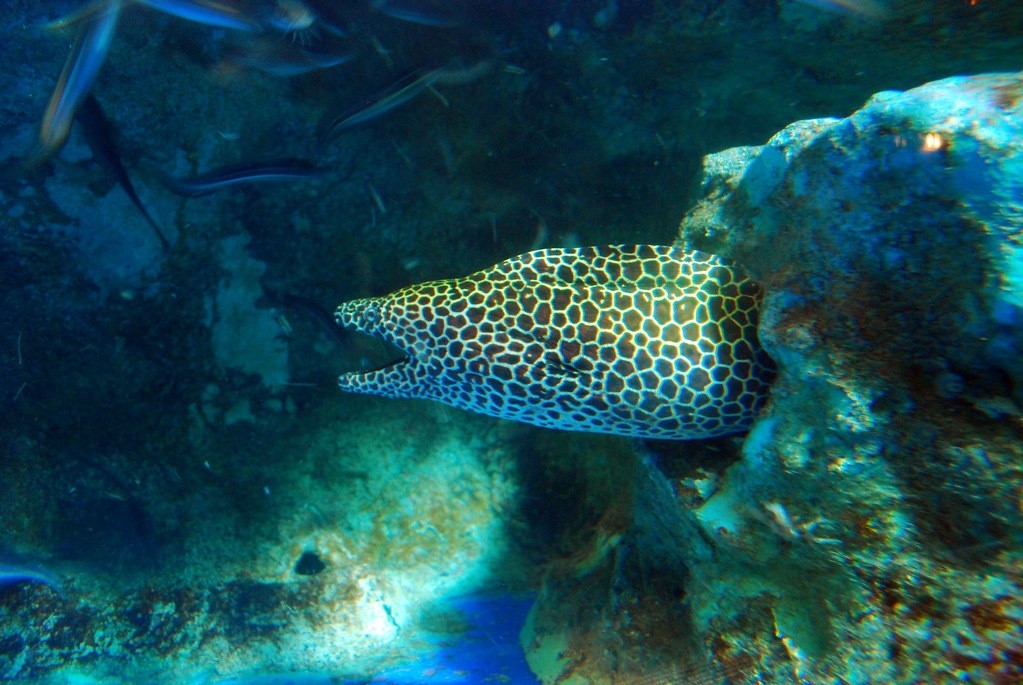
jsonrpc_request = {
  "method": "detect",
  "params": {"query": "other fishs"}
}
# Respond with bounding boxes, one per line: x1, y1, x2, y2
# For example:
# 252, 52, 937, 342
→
330, 243, 776, 442
0, 1, 1023, 603
